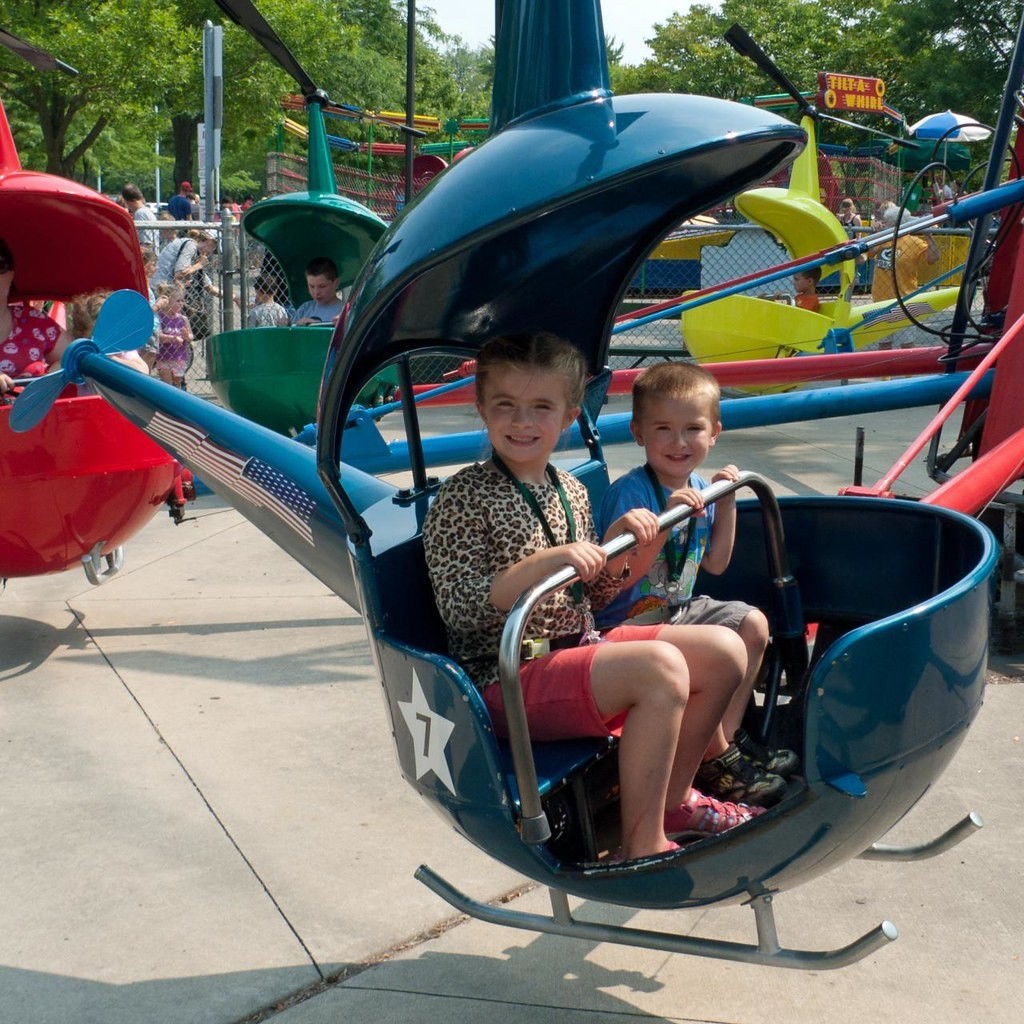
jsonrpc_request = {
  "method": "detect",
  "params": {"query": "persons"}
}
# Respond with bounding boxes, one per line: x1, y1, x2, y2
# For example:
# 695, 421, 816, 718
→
0, 239, 80, 399
759, 263, 822, 313
936, 175, 953, 199
598, 359, 796, 807
834, 198, 862, 241
245, 274, 291, 327
420, 328, 765, 863
69, 293, 155, 402
98, 180, 259, 386
872, 200, 901, 232
857, 207, 940, 302
291, 258, 349, 330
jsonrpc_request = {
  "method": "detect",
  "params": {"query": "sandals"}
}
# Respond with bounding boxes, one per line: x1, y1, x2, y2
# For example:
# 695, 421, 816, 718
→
664, 788, 767, 840
614, 841, 680, 861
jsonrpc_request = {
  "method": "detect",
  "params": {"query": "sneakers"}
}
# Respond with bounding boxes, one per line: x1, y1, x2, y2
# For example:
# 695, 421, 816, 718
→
694, 743, 787, 808
728, 728, 796, 778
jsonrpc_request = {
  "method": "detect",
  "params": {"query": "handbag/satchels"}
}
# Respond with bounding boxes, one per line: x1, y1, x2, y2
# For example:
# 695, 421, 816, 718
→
181, 297, 212, 341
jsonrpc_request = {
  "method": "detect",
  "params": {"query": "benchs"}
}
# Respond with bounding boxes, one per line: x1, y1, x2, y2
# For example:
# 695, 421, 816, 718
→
817, 300, 851, 322
371, 457, 614, 811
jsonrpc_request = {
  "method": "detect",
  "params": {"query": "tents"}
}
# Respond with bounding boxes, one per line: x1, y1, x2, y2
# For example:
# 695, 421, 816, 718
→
853, 138, 972, 175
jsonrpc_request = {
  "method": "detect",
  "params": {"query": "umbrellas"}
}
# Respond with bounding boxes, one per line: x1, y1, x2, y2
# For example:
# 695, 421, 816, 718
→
908, 110, 993, 185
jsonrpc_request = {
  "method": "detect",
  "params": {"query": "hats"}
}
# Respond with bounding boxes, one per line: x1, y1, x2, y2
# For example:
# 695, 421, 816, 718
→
205, 229, 223, 253
182, 182, 193, 191
